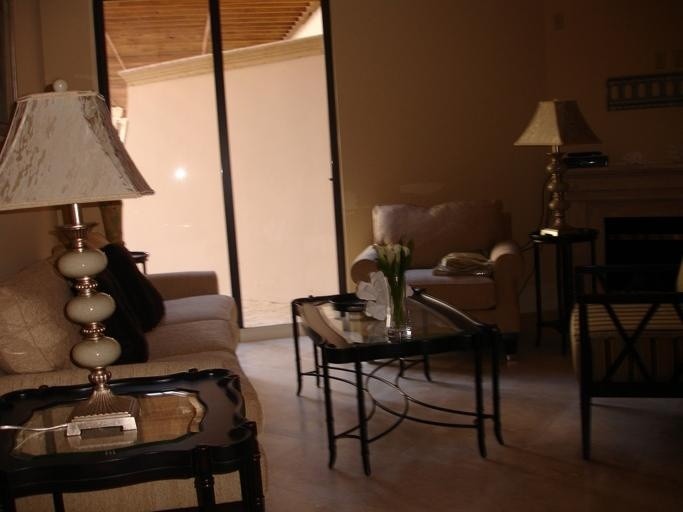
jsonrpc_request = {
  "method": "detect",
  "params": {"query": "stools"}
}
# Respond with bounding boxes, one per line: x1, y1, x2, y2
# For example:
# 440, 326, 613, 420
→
528, 225, 603, 352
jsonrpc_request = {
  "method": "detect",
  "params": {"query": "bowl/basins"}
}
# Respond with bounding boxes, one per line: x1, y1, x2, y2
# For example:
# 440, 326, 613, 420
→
328, 292, 366, 312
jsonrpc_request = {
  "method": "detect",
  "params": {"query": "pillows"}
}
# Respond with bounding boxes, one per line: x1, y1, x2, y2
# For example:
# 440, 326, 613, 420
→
89, 244, 166, 365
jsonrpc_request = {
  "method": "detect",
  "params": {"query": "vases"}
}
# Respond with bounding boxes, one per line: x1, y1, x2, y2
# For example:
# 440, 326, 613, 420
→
381, 275, 413, 339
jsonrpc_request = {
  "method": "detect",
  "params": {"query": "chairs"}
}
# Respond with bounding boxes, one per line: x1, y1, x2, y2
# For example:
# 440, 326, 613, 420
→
349, 197, 525, 363
570, 241, 683, 461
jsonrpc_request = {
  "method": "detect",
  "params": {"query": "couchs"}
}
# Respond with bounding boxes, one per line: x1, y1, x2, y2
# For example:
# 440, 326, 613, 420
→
1, 232, 265, 512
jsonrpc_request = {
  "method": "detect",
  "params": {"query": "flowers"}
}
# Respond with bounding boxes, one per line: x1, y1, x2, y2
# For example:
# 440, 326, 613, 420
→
368, 232, 416, 329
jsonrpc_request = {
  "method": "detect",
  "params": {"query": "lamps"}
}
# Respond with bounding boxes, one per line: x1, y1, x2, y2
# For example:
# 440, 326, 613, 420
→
513, 98, 602, 237
0, 78, 209, 458
